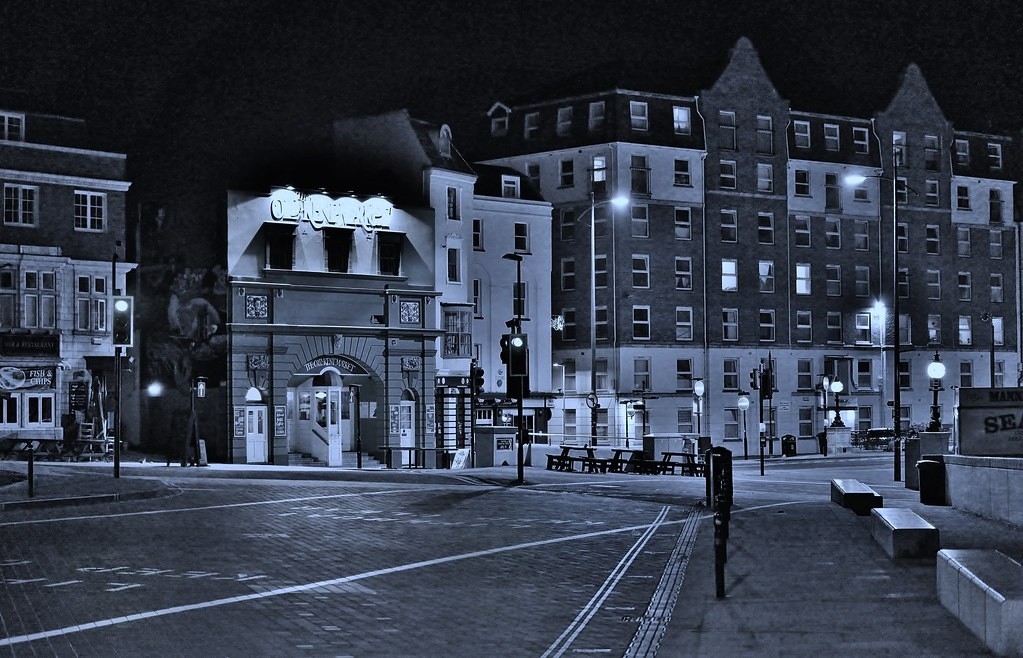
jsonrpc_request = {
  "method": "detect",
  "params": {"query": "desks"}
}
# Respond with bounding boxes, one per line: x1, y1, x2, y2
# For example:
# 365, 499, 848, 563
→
659, 451, 700, 477
609, 449, 649, 475
3, 439, 111, 463
555, 446, 599, 474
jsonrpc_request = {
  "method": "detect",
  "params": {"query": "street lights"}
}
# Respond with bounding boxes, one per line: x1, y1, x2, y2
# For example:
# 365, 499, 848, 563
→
829, 376, 846, 427
502, 252, 526, 485
112, 239, 123, 479
843, 150, 920, 481
927, 349, 947, 432
980, 310, 995, 388
577, 189, 631, 447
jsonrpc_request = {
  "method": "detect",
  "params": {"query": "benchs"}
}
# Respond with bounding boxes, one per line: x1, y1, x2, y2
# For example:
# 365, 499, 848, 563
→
937, 549, 1023, 658
76, 451, 106, 458
31, 450, 53, 456
870, 506, 940, 560
546, 454, 705, 477
831, 478, 884, 516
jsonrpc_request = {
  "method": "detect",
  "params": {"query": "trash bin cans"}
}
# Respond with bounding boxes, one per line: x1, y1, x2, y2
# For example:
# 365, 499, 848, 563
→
705, 446, 734, 507
915, 460, 946, 505
780, 435, 796, 457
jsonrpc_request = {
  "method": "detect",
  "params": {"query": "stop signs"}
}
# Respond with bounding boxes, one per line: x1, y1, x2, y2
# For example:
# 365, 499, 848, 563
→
738, 397, 749, 410
694, 380, 704, 396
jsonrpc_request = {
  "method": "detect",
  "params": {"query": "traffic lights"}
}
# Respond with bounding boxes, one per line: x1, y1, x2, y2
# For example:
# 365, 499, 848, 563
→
749, 368, 759, 390
111, 296, 134, 348
508, 334, 529, 377
474, 366, 486, 395
